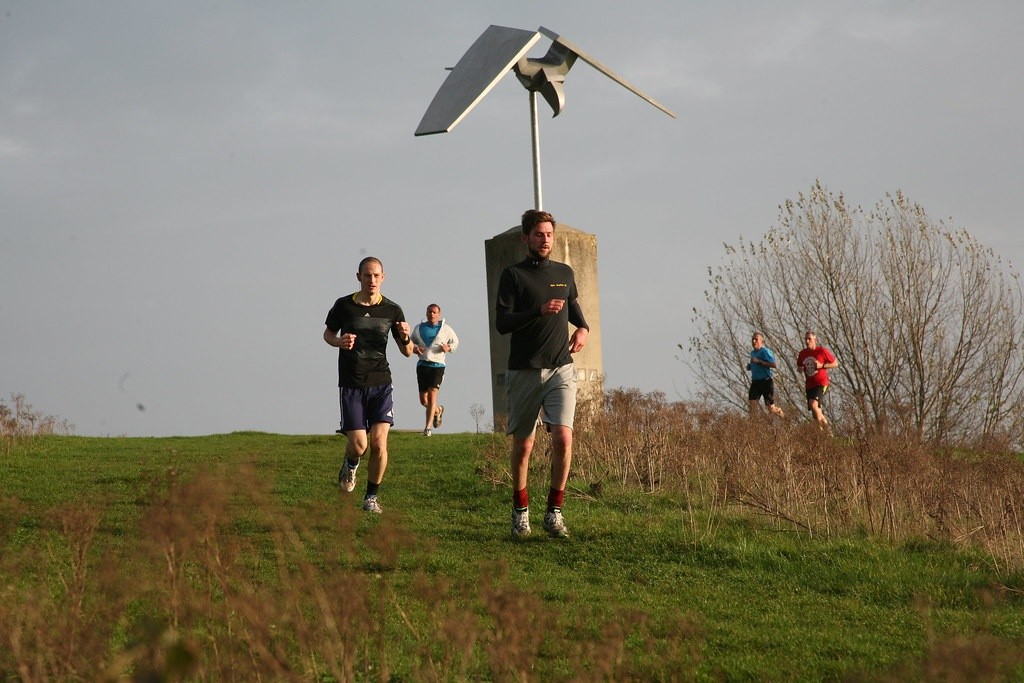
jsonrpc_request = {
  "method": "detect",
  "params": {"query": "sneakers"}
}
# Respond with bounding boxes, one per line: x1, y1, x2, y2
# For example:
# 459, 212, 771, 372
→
542, 508, 569, 540
433, 405, 444, 428
363, 495, 382, 514
512, 507, 531, 540
338, 454, 360, 493
424, 428, 432, 437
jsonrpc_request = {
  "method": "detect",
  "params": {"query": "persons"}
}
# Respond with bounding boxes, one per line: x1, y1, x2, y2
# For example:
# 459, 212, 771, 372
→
495, 210, 590, 537
410, 304, 458, 437
746, 332, 785, 419
797, 331, 838, 437
323, 257, 414, 514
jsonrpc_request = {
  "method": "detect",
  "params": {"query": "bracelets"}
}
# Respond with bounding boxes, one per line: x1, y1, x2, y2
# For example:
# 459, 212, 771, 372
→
822, 364, 825, 368
401, 336, 410, 345
759, 361, 762, 364
